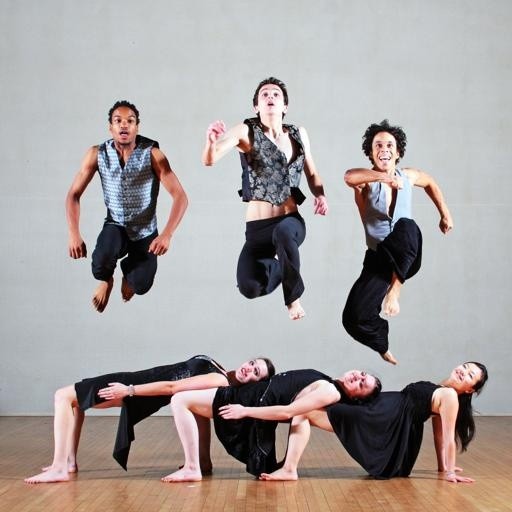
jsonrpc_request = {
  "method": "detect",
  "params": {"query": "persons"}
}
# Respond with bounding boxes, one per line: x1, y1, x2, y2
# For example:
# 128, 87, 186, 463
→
200, 79, 329, 321
65, 100, 190, 313
26, 355, 277, 485
161, 367, 383, 481
258, 361, 488, 483
341, 119, 454, 364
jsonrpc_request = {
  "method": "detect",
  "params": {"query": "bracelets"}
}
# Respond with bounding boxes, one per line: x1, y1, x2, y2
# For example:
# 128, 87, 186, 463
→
129, 384, 134, 397
